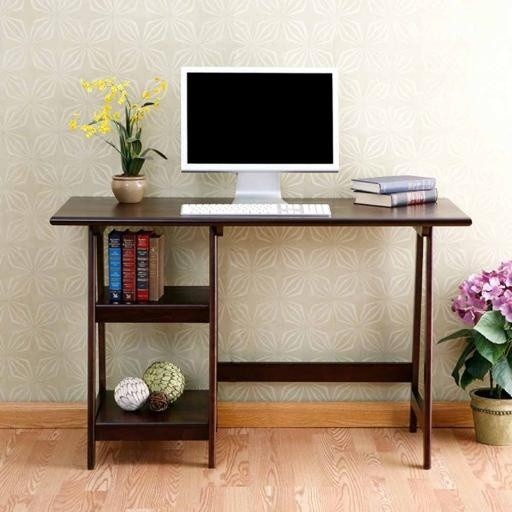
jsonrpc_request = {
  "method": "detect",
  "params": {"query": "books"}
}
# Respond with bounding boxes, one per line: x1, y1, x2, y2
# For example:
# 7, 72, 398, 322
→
349, 175, 439, 209
108, 229, 166, 305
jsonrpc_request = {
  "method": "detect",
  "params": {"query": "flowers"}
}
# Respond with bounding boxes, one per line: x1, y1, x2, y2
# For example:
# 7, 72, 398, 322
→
68, 74, 169, 178
436, 260, 512, 404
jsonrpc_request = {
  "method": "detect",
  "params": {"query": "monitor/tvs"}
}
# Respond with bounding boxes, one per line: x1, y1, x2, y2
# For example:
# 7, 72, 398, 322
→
179, 66, 339, 204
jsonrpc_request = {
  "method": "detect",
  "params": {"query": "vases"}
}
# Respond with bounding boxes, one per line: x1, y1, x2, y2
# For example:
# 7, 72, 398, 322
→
111, 176, 147, 204
467, 388, 512, 447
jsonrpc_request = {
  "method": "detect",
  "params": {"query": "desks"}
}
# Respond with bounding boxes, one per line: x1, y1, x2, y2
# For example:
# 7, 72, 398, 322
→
49, 196, 471, 468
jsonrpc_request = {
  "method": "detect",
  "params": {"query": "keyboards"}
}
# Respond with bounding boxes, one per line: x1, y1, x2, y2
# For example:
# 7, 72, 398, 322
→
180, 203, 331, 219
